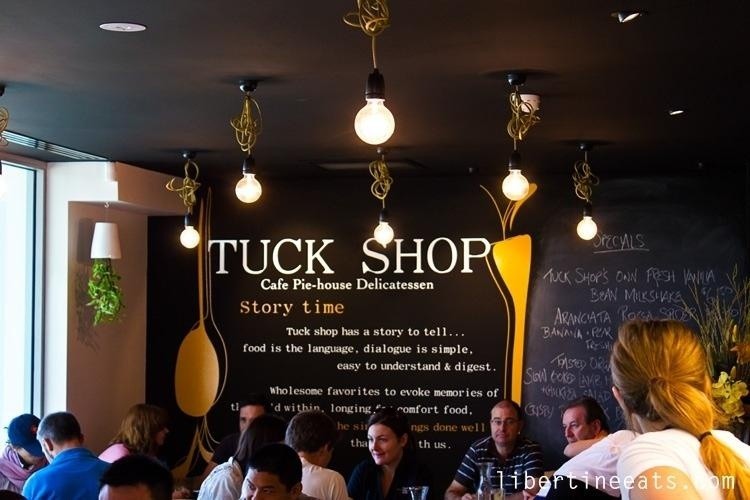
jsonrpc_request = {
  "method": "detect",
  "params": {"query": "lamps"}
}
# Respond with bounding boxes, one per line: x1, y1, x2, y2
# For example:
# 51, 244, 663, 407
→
500, 71, 543, 203
368, 145, 395, 247
343, 1, 397, 146
166, 149, 202, 249
230, 79, 264, 204
571, 143, 599, 241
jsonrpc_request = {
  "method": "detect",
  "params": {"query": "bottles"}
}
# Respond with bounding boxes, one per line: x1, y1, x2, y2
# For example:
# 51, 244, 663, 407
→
475, 462, 494, 500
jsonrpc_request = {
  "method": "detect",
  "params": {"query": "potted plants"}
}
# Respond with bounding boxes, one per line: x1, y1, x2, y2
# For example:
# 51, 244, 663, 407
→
86, 221, 128, 327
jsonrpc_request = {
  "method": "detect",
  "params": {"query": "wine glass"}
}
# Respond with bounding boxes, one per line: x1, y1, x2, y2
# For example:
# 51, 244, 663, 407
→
408, 486, 429, 500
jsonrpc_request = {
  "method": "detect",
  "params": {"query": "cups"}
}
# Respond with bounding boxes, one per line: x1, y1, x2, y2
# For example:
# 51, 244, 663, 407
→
543, 471, 555, 481
494, 488, 506, 500
524, 478, 540, 496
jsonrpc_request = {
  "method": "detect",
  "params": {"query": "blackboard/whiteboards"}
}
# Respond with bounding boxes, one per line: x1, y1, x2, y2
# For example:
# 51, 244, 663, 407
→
520, 199, 750, 473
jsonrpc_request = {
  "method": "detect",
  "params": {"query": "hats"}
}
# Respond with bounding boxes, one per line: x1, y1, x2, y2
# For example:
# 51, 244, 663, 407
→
8, 414, 45, 457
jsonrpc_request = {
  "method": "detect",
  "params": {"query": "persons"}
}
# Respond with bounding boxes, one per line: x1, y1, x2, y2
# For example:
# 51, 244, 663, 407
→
0, 322, 750, 499
